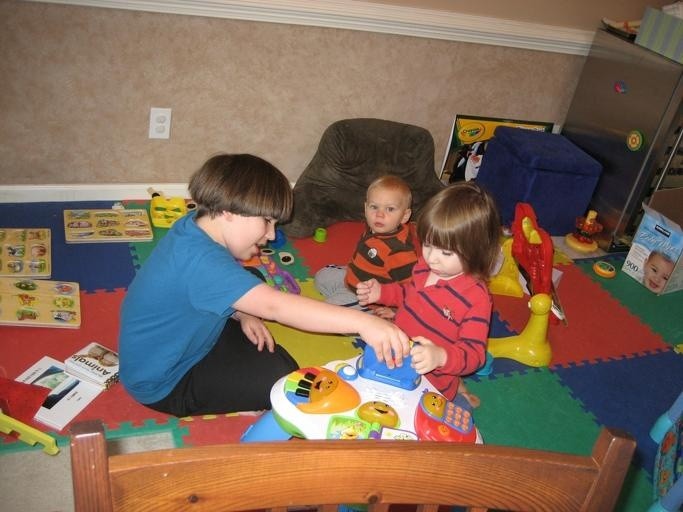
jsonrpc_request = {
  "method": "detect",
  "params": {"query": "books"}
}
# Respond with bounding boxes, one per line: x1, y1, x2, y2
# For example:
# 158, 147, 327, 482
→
13, 356, 103, 430
64, 341, 119, 389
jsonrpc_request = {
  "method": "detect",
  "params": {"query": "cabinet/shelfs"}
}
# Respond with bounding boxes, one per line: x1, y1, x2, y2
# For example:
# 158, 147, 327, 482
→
561, 28, 683, 254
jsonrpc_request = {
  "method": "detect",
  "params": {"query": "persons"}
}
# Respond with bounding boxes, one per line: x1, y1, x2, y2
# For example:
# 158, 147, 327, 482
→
314, 174, 419, 321
356, 179, 500, 402
642, 251, 674, 294
117, 152, 411, 417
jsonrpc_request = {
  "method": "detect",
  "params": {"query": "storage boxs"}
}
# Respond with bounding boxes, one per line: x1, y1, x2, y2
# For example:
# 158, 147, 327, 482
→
634, 6, 683, 65
621, 188, 683, 297
476, 126, 603, 236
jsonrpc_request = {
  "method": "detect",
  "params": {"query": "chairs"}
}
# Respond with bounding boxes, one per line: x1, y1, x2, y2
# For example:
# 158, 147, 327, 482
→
67, 416, 637, 512
